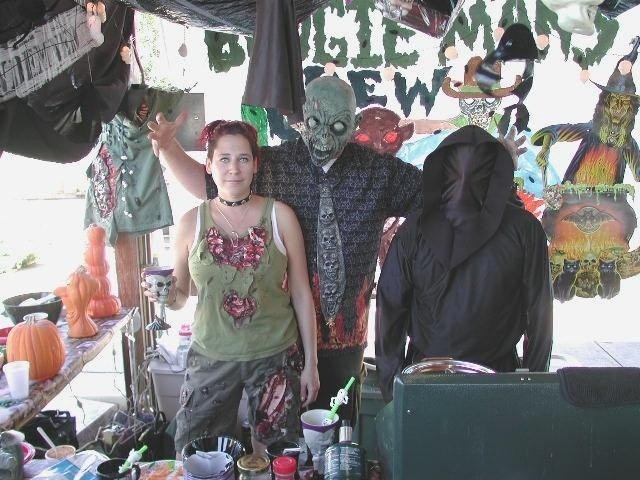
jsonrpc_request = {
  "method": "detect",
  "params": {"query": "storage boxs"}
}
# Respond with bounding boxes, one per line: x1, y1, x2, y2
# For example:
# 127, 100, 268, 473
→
147, 352, 251, 432
43, 396, 118, 450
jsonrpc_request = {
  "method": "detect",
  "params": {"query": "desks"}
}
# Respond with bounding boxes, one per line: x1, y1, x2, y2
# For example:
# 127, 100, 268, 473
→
1, 300, 141, 440
2, 434, 373, 480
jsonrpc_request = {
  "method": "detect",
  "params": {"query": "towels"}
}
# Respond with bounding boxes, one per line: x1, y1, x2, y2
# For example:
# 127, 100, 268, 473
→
557, 365, 640, 410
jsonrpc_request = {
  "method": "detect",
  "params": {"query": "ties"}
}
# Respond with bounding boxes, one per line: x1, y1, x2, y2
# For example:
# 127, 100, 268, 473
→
316, 179, 347, 326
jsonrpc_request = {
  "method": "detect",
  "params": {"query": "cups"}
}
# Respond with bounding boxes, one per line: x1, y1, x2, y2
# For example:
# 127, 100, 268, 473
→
44, 445, 76, 467
2, 360, 30, 398
266, 441, 299, 480
95, 459, 141, 480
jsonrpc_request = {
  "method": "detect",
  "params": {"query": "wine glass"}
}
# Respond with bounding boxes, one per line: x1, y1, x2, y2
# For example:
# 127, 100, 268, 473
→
141, 266, 176, 331
301, 409, 340, 478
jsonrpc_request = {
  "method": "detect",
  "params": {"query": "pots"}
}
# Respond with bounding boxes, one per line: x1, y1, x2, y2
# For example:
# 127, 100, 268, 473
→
403, 356, 495, 375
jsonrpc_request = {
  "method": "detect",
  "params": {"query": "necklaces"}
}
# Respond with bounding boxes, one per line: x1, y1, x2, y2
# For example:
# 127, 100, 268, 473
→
212, 199, 250, 247
217, 192, 253, 206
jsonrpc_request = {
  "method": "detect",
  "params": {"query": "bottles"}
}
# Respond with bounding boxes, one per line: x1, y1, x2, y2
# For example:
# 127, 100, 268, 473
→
0, 431, 27, 480
177, 321, 203, 366
236, 454, 271, 480
326, 420, 365, 480
273, 456, 297, 479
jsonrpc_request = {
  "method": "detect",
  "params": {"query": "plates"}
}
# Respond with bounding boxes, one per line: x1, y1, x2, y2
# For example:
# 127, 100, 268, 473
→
21, 441, 34, 466
180, 436, 245, 480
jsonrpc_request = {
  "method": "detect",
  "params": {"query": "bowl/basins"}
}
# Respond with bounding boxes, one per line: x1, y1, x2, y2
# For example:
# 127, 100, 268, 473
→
2, 292, 63, 328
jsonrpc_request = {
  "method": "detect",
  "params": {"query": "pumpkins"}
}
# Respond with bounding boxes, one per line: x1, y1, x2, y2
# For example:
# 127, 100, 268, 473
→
6, 316, 66, 384
54, 225, 124, 338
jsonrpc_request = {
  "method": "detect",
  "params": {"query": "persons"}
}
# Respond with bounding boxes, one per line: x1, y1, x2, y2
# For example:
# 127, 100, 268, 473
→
374, 124, 553, 403
142, 120, 321, 459
148, 75, 528, 445
